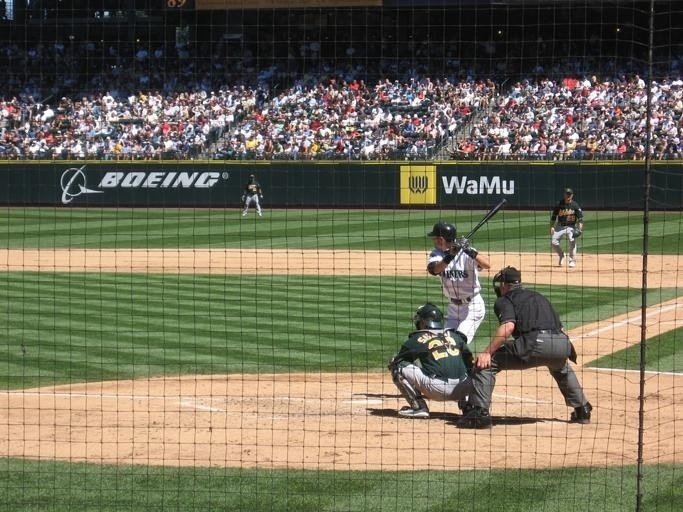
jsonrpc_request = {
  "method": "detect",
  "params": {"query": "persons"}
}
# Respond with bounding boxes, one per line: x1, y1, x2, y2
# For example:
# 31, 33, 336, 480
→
548, 188, 584, 267
388, 302, 475, 418
456, 266, 592, 428
0, 28, 683, 162
426, 223, 490, 345
241, 174, 264, 217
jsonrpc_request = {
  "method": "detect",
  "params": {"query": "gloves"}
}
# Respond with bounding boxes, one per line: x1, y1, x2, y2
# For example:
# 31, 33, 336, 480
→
459, 237, 478, 259
442, 243, 462, 264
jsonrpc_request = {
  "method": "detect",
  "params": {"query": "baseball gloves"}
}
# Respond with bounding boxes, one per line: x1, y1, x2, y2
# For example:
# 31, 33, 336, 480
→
572, 225, 583, 239
241, 194, 246, 202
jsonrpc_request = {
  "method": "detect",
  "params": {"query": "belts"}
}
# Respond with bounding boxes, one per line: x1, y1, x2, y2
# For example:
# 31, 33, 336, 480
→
451, 296, 471, 305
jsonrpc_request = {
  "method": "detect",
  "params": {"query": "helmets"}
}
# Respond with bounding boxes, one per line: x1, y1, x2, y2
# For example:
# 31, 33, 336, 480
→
427, 221, 457, 242
413, 303, 443, 329
563, 188, 575, 194
493, 265, 521, 297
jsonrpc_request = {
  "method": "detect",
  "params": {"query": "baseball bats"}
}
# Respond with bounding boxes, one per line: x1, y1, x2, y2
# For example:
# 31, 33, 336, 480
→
466, 198, 507, 239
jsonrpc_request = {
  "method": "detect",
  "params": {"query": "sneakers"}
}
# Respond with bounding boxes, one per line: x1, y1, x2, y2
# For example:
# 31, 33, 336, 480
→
559, 251, 567, 264
397, 408, 430, 419
455, 407, 490, 427
569, 412, 591, 424
568, 258, 576, 267
